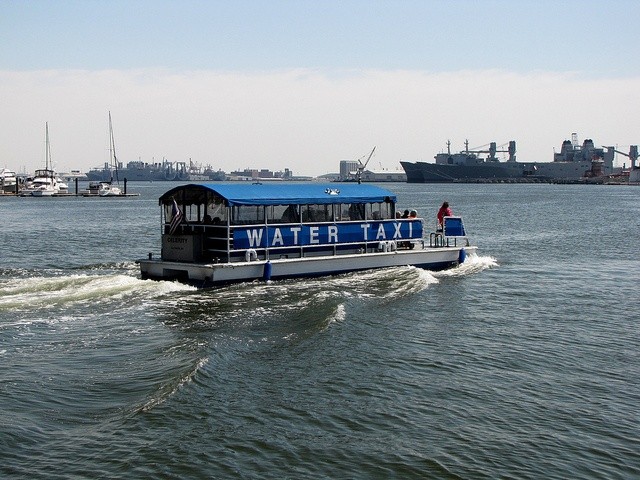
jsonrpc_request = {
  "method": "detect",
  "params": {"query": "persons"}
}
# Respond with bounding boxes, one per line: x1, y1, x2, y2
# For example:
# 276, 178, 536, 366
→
396, 209, 417, 249
372, 211, 382, 220
281, 205, 299, 223
437, 201, 452, 228
348, 203, 364, 221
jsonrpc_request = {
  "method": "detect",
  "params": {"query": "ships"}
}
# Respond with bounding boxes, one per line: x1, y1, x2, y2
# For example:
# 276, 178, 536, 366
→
399, 139, 614, 183
86, 157, 190, 181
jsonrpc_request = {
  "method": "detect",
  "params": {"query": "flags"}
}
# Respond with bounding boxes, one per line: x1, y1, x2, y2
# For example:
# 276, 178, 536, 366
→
169, 200, 183, 236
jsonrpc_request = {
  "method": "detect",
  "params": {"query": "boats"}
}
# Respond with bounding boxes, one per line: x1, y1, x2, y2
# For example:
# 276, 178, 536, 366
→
135, 184, 478, 288
0, 168, 139, 197
